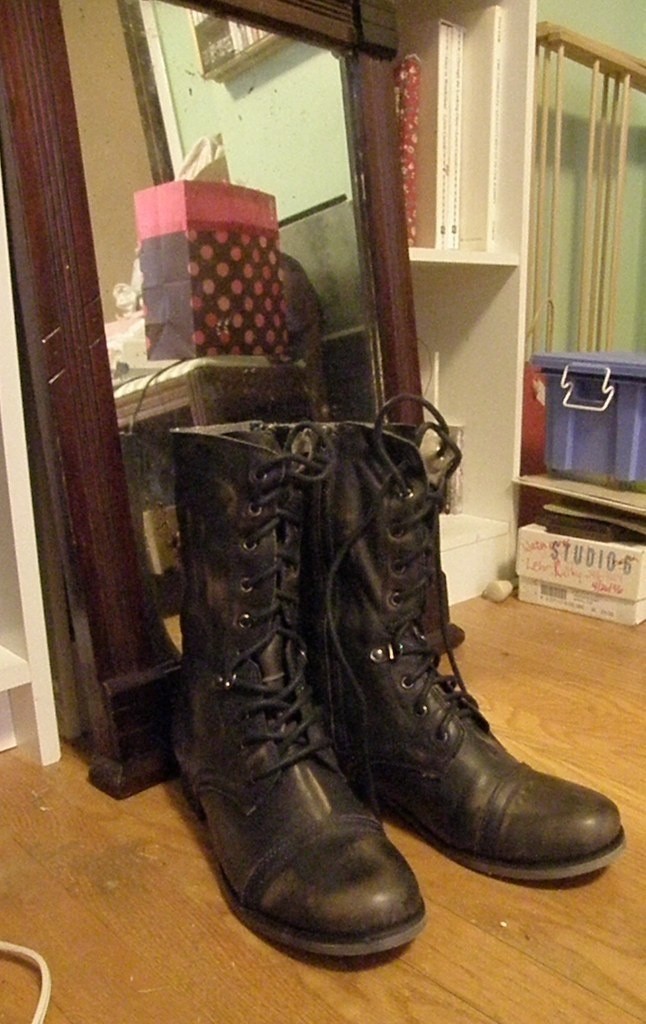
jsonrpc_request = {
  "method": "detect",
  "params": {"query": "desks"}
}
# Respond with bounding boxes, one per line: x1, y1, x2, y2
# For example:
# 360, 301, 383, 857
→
112, 363, 312, 568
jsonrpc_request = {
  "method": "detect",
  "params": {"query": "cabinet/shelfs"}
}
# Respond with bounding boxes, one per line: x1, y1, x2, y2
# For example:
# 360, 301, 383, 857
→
189, 8, 296, 83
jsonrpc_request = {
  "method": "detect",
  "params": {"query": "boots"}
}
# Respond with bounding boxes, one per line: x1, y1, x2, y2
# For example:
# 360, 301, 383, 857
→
307, 393, 626, 878
158, 417, 430, 955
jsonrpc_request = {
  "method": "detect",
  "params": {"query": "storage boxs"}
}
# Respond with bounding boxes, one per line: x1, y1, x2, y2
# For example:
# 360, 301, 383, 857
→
532, 351, 646, 483
514, 522, 646, 626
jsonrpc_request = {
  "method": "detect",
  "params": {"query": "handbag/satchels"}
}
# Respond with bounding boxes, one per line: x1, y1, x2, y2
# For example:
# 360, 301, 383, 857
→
133, 137, 288, 361
